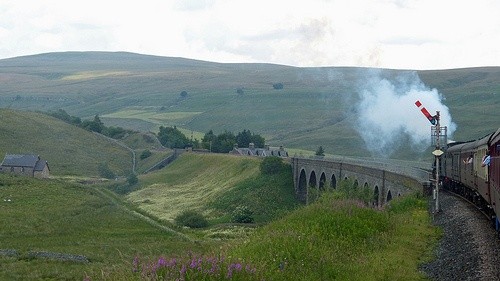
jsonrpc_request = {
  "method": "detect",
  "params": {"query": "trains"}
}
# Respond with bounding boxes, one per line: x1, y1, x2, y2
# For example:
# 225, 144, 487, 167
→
432, 129, 500, 226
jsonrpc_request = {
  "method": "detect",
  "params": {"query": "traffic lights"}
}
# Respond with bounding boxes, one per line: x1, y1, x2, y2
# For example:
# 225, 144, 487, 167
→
428, 115, 435, 126
